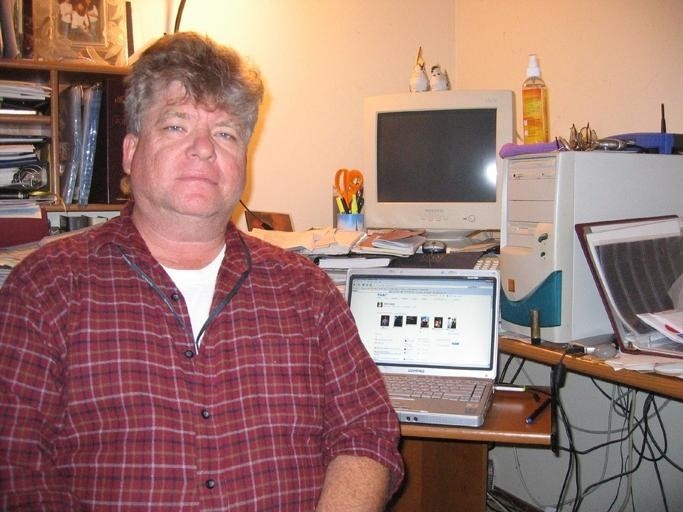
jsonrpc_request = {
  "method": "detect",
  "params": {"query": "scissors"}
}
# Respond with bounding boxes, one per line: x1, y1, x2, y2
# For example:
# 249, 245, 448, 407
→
334, 168, 363, 210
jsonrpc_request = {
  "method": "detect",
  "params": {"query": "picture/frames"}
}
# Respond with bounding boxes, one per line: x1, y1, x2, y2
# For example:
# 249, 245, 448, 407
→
22, 0, 134, 59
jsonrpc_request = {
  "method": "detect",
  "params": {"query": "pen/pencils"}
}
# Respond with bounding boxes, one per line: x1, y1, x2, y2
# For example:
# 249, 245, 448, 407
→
336, 187, 364, 214
526, 398, 551, 424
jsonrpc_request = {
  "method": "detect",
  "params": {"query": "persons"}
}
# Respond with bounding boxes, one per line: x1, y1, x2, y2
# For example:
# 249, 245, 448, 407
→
0, 32, 407, 512
58, 0, 101, 44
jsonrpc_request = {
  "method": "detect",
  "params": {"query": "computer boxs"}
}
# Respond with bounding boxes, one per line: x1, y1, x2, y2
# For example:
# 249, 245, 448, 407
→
497, 149, 682, 346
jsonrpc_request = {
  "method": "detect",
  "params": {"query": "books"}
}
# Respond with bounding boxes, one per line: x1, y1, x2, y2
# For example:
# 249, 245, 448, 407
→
574, 213, 682, 358
0, 78, 111, 293
0, 0, 25, 59
249, 227, 425, 268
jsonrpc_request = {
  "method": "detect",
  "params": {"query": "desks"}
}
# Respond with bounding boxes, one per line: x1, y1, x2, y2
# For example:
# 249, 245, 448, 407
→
387, 382, 560, 512
498, 337, 683, 512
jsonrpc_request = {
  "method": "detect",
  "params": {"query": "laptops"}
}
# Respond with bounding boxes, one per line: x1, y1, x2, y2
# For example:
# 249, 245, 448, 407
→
344, 269, 500, 428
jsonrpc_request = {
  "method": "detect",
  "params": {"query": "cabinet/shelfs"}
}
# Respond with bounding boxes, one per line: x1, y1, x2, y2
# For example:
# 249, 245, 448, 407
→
0, 58, 132, 211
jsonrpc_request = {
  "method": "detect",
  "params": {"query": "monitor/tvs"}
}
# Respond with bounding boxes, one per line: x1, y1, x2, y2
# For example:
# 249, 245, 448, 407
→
362, 90, 516, 242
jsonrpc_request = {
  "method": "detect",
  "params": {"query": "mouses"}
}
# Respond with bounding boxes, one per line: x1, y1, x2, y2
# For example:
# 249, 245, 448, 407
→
422, 242, 446, 253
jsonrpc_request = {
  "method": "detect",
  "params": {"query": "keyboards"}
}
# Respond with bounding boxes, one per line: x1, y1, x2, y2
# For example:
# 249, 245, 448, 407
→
473, 256, 499, 269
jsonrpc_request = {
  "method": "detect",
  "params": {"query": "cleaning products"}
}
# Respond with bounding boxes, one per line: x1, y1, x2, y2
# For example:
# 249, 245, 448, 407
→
522, 54, 550, 144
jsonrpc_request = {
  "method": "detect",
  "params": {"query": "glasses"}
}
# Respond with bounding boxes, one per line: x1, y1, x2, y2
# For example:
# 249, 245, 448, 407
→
569, 121, 599, 152
556, 135, 570, 150
